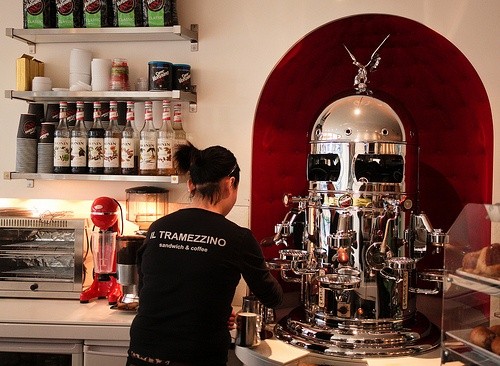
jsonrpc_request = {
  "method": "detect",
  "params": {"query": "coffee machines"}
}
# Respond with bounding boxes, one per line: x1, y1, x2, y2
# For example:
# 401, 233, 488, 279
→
116, 186, 170, 305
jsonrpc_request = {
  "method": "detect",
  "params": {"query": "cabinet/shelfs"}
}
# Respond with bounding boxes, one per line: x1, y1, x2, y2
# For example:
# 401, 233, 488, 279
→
2, 23, 199, 190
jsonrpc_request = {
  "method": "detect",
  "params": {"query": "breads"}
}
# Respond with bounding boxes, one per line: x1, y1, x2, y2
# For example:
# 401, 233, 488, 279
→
471, 324, 500, 357
461, 242, 500, 277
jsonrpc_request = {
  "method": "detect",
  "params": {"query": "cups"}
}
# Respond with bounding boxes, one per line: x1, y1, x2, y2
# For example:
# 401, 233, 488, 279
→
236, 312, 258, 347
110, 64, 129, 92
15, 101, 127, 173
243, 296, 264, 332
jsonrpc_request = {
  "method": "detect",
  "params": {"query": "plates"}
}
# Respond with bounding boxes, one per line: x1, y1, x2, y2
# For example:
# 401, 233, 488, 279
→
456, 267, 500, 286
445, 328, 500, 364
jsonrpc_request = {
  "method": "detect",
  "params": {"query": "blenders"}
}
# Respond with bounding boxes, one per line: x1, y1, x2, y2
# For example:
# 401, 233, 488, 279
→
78, 197, 123, 306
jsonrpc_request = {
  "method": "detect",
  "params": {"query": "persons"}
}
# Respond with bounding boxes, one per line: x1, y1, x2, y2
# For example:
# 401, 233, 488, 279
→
126, 140, 283, 366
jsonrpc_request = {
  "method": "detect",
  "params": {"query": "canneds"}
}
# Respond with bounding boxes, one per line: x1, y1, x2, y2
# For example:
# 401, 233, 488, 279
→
111, 59, 129, 91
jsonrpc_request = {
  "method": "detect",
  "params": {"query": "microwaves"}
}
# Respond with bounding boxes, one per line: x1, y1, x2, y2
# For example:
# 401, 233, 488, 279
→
0, 218, 90, 300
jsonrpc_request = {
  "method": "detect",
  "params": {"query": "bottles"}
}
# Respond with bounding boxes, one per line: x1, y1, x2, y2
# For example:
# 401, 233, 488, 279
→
138, 101, 157, 176
87, 102, 105, 174
104, 101, 122, 175
148, 61, 191, 94
156, 99, 175, 175
70, 101, 87, 174
52, 102, 70, 174
120, 101, 138, 176
172, 104, 186, 175
